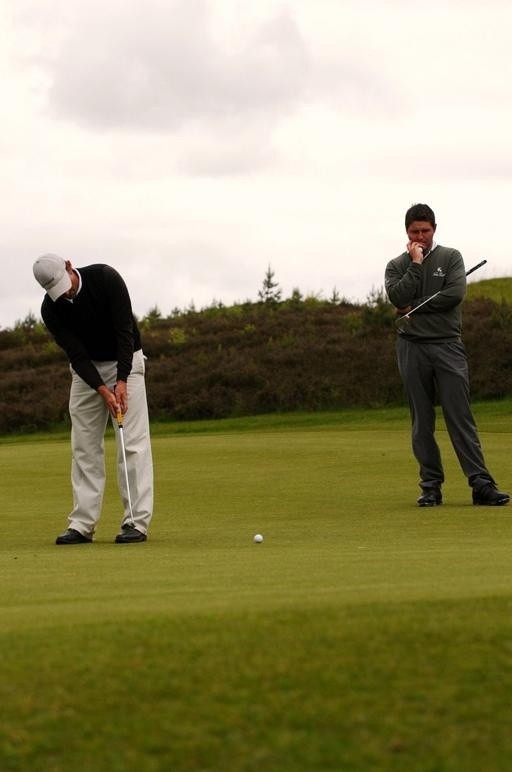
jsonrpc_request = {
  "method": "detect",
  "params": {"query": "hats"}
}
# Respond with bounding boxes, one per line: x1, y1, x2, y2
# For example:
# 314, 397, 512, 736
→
33, 253, 72, 302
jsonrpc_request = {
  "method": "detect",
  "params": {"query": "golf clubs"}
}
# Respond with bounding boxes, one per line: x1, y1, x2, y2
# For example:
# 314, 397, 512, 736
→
391, 260, 487, 335
114, 385, 135, 530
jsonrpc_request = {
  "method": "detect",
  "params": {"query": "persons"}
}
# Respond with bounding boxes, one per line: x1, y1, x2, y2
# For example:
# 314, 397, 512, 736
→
386, 203, 509, 506
33, 254, 154, 544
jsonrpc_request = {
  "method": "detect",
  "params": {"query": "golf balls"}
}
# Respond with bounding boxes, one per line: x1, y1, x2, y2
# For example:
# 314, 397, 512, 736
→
254, 534, 263, 543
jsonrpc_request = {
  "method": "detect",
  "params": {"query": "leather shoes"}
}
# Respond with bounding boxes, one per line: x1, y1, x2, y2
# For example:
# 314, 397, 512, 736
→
56, 528, 91, 545
115, 526, 147, 543
472, 485, 509, 506
416, 489, 443, 506
